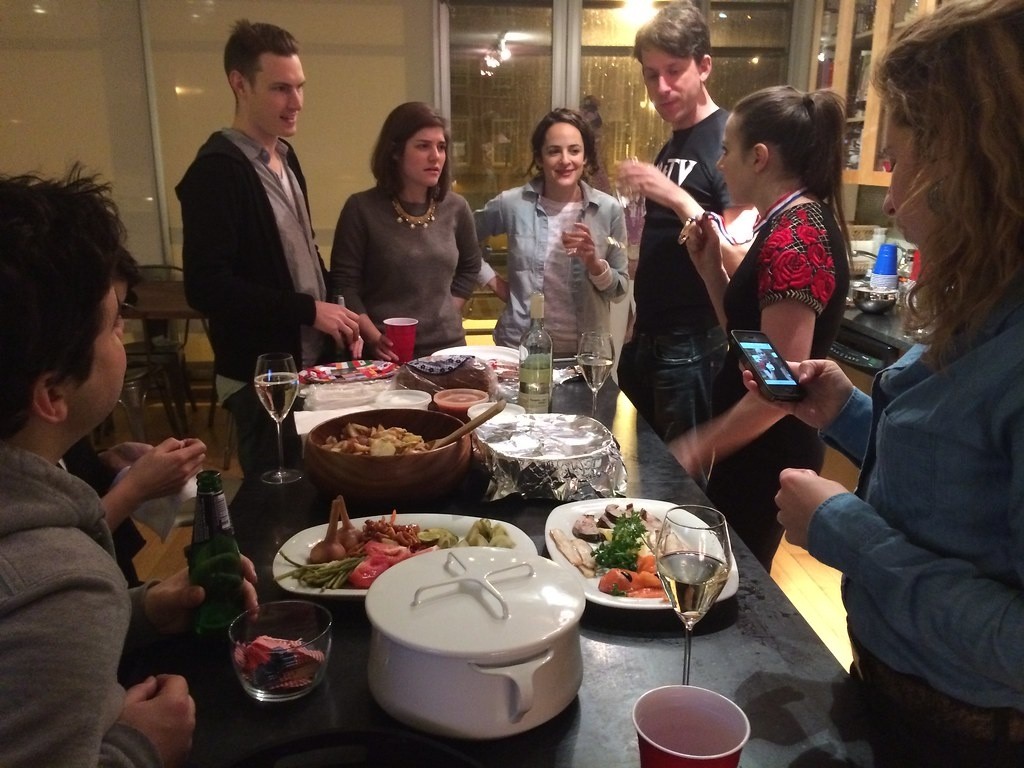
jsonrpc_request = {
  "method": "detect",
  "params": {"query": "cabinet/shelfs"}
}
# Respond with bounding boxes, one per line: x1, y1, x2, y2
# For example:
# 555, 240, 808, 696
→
808, 0, 954, 186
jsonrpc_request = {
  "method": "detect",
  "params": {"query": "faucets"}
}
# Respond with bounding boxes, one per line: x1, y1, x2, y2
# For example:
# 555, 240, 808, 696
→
850, 241, 907, 268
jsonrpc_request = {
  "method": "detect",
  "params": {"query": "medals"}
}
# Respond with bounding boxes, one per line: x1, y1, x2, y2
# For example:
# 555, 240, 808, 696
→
678, 221, 690, 244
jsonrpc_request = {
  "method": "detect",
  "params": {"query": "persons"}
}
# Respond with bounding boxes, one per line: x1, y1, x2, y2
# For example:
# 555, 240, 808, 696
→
330, 101, 481, 363
672, 86, 856, 577
755, 348, 788, 380
57, 246, 206, 588
472, 108, 628, 354
617, 0, 758, 488
0, 175, 259, 768
738, 1, 1024, 768
173, 22, 361, 486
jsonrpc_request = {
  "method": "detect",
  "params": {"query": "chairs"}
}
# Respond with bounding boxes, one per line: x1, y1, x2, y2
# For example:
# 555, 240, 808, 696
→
90, 261, 217, 451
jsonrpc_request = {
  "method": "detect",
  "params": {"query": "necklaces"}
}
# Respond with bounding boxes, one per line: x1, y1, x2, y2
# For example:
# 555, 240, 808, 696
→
392, 197, 436, 229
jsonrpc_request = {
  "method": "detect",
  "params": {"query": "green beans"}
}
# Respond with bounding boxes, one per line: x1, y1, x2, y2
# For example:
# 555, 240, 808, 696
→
276, 550, 361, 592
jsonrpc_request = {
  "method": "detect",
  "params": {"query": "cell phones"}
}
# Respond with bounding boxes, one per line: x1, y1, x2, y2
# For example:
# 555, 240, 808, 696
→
728, 329, 807, 402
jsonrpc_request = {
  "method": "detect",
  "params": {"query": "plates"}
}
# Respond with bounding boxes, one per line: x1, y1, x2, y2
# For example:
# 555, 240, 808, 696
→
273, 514, 539, 603
545, 497, 739, 609
298, 360, 400, 384
430, 345, 520, 365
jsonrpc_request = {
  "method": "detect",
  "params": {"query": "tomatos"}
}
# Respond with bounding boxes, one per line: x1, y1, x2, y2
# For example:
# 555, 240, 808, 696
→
350, 540, 440, 590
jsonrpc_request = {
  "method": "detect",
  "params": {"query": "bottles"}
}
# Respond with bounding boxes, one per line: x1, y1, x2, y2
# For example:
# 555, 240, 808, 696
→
188, 469, 246, 647
518, 291, 553, 414
332, 294, 352, 360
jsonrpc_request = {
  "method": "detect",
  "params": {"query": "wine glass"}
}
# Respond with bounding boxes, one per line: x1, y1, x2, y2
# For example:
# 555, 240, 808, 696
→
655, 504, 732, 686
254, 353, 303, 484
556, 215, 589, 293
577, 331, 616, 420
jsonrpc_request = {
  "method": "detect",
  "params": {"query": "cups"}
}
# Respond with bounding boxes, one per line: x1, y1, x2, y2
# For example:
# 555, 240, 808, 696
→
632, 685, 751, 768
228, 600, 333, 702
383, 317, 419, 367
872, 245, 897, 289
377, 389, 526, 422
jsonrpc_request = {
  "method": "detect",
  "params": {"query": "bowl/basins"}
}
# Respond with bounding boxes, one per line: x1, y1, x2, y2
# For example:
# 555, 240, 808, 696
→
851, 287, 898, 313
302, 409, 474, 513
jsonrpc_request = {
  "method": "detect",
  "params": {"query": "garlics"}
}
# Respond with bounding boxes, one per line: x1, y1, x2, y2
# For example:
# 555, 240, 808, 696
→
310, 495, 361, 562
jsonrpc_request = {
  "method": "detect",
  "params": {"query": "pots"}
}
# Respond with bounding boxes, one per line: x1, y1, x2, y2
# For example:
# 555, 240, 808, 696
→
365, 545, 587, 741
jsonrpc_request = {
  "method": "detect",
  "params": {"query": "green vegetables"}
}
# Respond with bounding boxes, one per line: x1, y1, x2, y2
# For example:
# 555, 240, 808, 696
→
590, 510, 651, 597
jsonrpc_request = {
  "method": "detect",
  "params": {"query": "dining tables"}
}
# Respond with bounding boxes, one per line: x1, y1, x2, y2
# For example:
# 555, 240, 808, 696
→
122, 359, 934, 768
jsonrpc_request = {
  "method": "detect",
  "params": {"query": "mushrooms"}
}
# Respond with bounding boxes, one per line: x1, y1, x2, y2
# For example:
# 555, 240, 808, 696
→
571, 503, 636, 542
